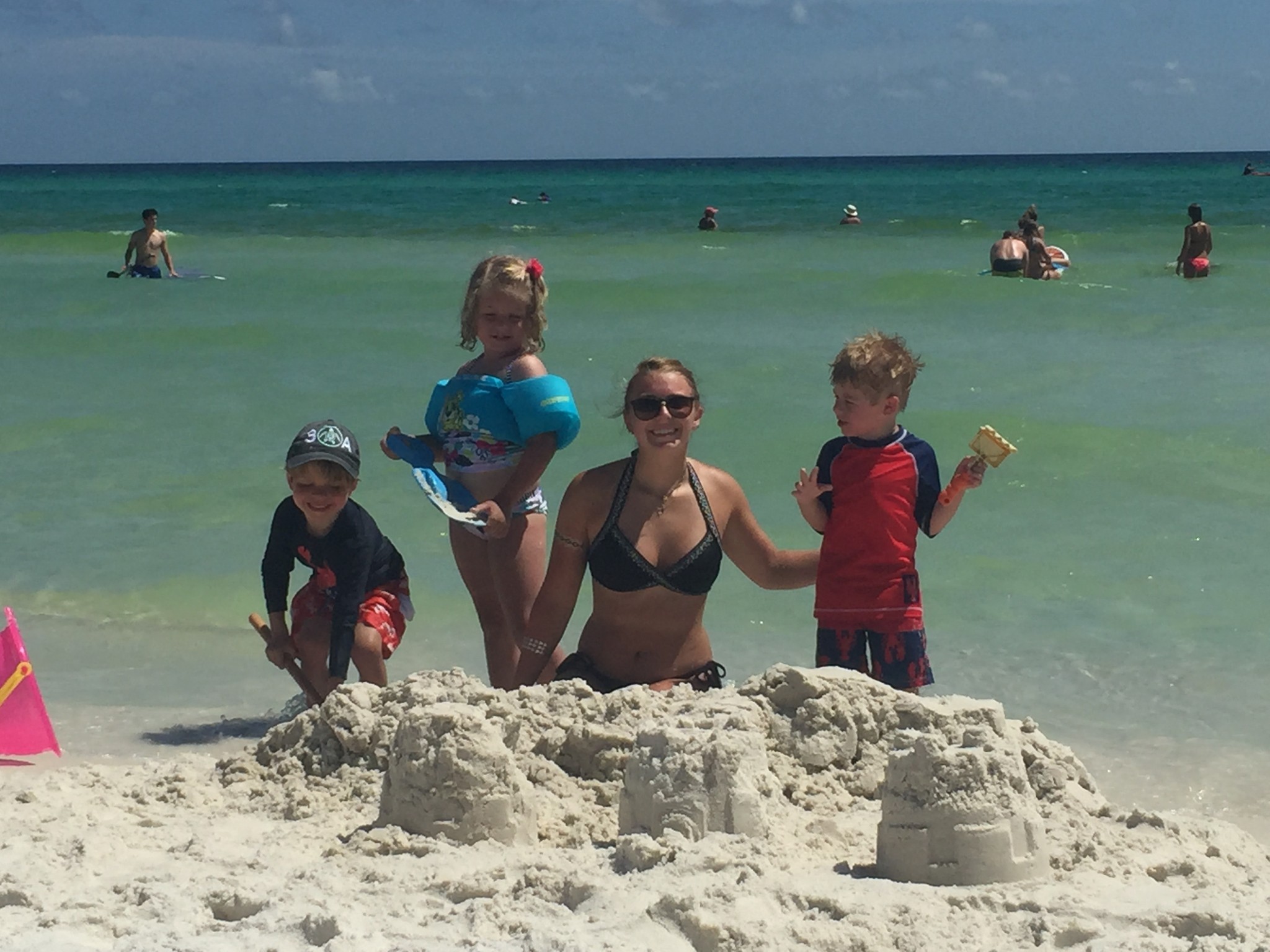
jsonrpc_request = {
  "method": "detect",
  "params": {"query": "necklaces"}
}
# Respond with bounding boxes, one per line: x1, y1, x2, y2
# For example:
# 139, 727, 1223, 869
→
632, 465, 690, 514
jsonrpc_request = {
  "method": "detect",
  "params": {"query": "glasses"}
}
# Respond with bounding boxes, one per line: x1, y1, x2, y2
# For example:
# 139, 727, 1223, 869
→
627, 394, 696, 420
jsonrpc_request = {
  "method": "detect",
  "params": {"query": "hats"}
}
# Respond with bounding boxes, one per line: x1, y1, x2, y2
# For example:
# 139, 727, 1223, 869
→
285, 420, 360, 481
843, 204, 858, 216
705, 207, 718, 214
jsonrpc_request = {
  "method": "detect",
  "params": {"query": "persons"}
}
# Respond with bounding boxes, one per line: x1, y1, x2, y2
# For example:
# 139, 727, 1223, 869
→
1240, 162, 1269, 177
539, 192, 552, 202
699, 206, 719, 232
1175, 203, 1212, 279
379, 255, 566, 692
989, 203, 1062, 280
260, 419, 411, 709
840, 205, 861, 225
122, 208, 183, 280
792, 329, 989, 695
509, 358, 821, 699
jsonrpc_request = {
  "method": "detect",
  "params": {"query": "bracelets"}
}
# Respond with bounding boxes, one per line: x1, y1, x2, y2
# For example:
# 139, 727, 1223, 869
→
519, 636, 552, 660
